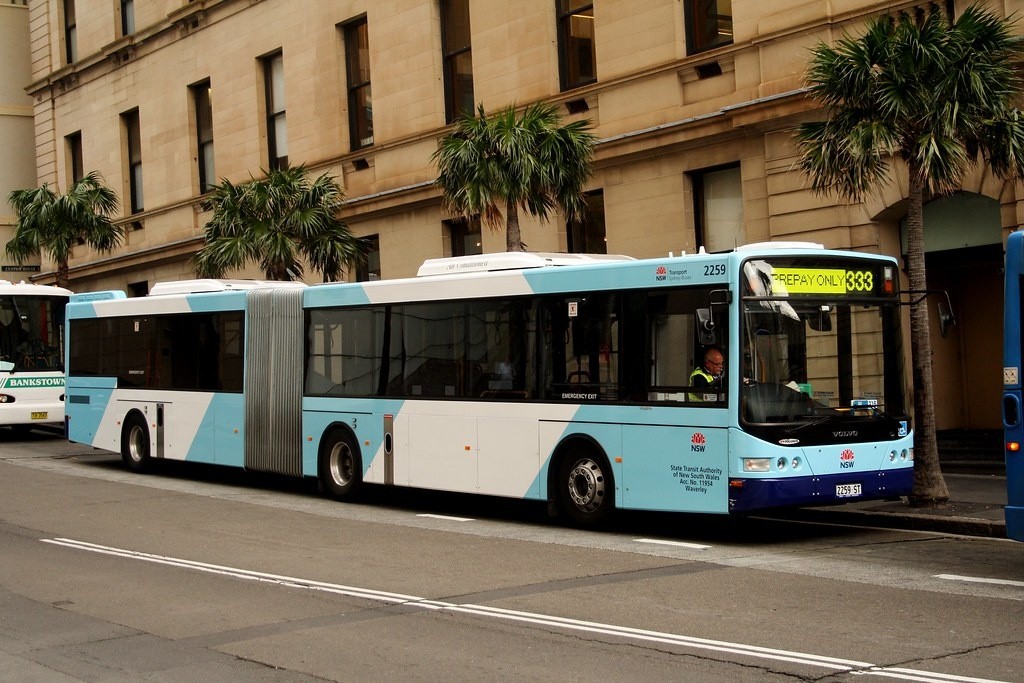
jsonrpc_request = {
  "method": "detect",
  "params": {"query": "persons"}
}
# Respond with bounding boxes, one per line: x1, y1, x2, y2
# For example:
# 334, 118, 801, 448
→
687, 349, 750, 402
743, 351, 753, 381
16, 328, 55, 370
496, 351, 517, 386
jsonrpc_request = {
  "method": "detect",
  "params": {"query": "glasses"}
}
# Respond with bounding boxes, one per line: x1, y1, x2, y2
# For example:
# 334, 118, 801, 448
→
707, 358, 724, 367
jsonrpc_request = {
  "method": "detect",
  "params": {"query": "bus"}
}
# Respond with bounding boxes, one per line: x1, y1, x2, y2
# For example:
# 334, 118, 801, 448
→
994, 230, 1024, 542
65, 238, 957, 523
0, 278, 75, 435
